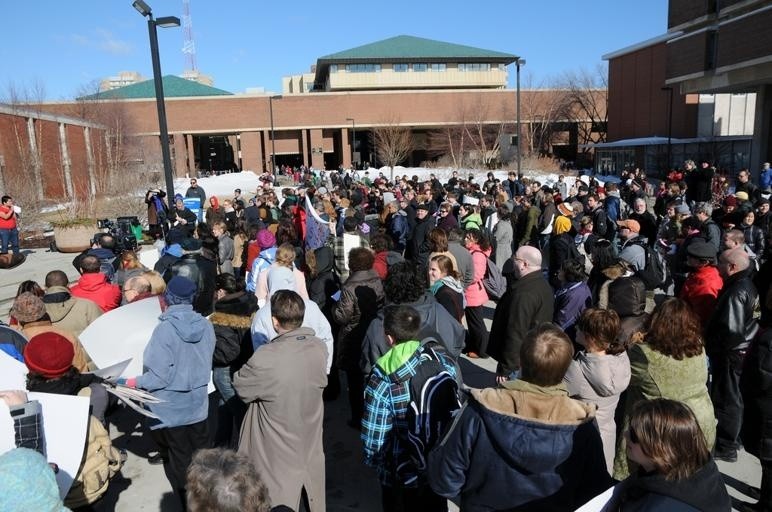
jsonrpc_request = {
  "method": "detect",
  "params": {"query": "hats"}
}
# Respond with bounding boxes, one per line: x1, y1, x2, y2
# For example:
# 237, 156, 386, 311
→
557, 202, 573, 216
632, 178, 642, 187
736, 191, 749, 200
688, 242, 716, 259
167, 277, 196, 299
723, 196, 737, 205
11, 292, 47, 322
256, 230, 275, 247
616, 219, 640, 232
24, 332, 75, 377
416, 205, 428, 210
182, 238, 201, 250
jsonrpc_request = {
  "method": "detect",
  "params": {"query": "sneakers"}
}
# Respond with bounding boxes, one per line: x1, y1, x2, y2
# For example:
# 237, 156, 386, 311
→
469, 352, 479, 357
713, 447, 738, 462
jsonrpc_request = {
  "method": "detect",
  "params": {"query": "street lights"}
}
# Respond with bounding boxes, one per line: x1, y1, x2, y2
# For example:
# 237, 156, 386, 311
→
130, 1, 182, 227
504, 56, 525, 181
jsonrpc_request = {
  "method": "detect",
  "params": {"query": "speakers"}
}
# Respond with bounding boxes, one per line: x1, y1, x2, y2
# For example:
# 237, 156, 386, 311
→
117, 216, 137, 236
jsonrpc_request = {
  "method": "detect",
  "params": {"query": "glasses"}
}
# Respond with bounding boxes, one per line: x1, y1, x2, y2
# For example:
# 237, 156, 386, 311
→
440, 210, 447, 213
629, 425, 640, 444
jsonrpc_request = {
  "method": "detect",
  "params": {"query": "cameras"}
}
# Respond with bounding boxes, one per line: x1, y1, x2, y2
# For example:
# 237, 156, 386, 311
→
153, 192, 158, 196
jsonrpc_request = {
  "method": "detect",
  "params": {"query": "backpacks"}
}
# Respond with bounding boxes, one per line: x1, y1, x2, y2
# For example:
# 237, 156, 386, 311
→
557, 235, 585, 265
474, 221, 496, 252
386, 348, 463, 487
626, 239, 671, 289
63, 387, 123, 510
471, 250, 509, 300
593, 207, 617, 234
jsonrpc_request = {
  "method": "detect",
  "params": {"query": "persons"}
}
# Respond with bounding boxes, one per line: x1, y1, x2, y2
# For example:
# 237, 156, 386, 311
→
0, 159, 772, 512
